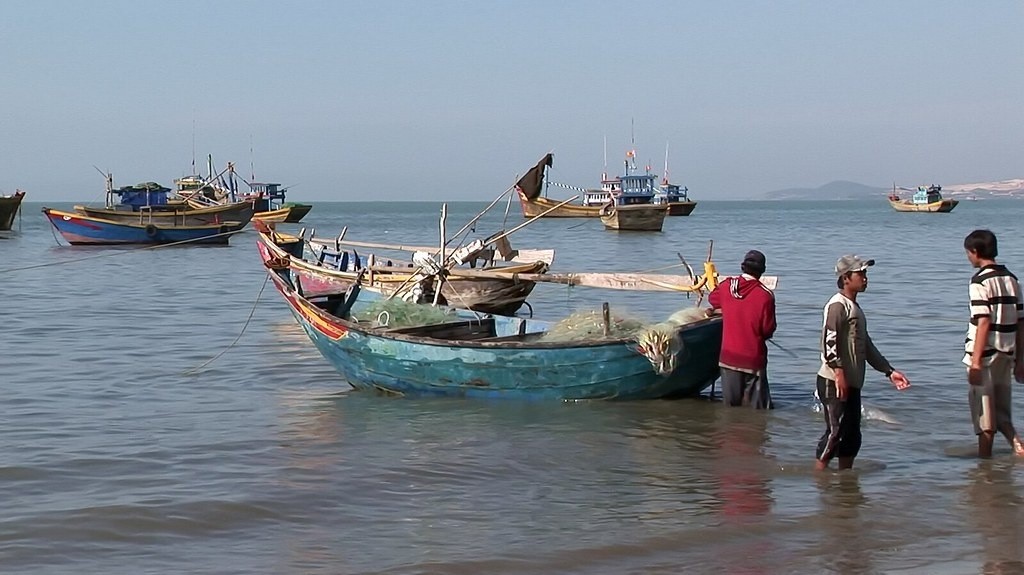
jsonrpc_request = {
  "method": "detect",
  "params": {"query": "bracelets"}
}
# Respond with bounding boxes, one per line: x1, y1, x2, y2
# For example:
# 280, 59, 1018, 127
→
886, 367, 895, 377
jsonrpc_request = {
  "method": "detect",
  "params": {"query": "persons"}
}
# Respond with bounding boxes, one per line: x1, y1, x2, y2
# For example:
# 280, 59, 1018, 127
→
708, 250, 778, 410
962, 230, 1024, 459
815, 255, 910, 469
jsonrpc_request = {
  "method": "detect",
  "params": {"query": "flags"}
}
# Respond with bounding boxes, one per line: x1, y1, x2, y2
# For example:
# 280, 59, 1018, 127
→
627, 151, 634, 156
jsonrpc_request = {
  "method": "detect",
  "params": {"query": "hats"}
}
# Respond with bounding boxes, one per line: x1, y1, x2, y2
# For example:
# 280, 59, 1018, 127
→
744, 250, 766, 267
835, 254, 875, 277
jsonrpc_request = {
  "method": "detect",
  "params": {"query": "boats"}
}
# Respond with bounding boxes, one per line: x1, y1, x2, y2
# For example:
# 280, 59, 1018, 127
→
513, 148, 697, 218
0, 189, 31, 241
249, 204, 783, 402
40, 153, 314, 244
352, 254, 547, 317
598, 157, 667, 231
887, 180, 960, 213
